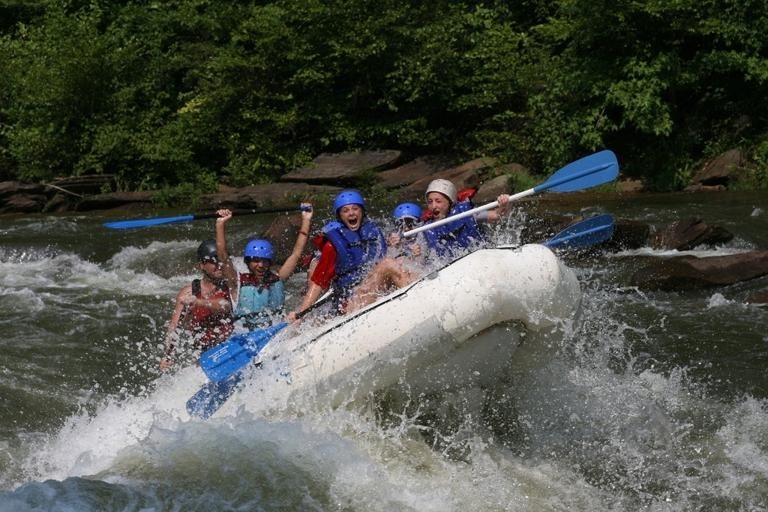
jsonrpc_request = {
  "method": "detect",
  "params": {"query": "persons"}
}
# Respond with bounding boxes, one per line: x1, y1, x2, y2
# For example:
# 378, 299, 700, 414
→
161, 178, 511, 371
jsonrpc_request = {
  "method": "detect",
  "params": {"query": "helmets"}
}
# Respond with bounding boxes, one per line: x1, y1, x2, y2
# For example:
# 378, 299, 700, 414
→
393, 203, 420, 222
333, 191, 365, 214
244, 239, 273, 260
198, 239, 218, 261
426, 178, 458, 205
321, 220, 343, 233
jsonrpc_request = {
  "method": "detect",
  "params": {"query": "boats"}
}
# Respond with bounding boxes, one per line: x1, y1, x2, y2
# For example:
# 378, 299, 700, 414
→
144, 242, 583, 440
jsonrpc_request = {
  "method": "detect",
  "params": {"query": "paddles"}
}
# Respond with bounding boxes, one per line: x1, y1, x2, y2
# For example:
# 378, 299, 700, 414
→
399, 150, 619, 238
103, 204, 312, 229
545, 213, 615, 248
186, 288, 336, 419
199, 247, 416, 385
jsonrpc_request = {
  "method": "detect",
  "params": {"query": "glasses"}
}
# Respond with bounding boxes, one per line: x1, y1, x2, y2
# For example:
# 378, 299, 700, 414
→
203, 256, 217, 263
